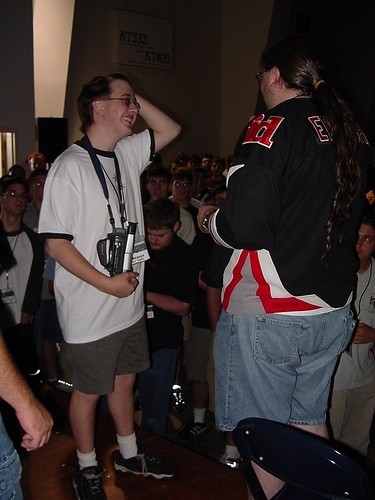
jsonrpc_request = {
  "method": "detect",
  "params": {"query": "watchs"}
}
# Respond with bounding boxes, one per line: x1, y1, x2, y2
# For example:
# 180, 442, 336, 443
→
200, 208, 217, 231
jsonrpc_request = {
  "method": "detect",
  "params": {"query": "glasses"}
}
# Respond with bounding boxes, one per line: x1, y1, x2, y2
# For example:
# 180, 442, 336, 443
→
95, 97, 141, 110
255, 69, 270, 82
2, 191, 30, 199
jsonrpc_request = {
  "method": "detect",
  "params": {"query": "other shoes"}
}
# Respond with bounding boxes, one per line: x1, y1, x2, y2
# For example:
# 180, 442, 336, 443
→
28, 365, 244, 471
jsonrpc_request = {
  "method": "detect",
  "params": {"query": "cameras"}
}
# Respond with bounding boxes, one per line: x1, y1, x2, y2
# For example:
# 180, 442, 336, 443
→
97, 227, 127, 277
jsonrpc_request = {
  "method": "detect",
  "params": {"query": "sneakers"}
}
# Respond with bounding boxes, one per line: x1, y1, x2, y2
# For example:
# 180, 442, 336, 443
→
72, 461, 107, 500
113, 449, 178, 480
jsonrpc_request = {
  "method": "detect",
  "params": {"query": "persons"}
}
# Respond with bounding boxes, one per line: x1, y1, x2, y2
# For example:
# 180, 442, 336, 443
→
194, 41, 365, 500
132, 198, 202, 475
0, 336, 54, 500
0, 152, 238, 237
35, 70, 182, 500
202, 240, 245, 471
321, 202, 375, 453
1, 176, 46, 359
